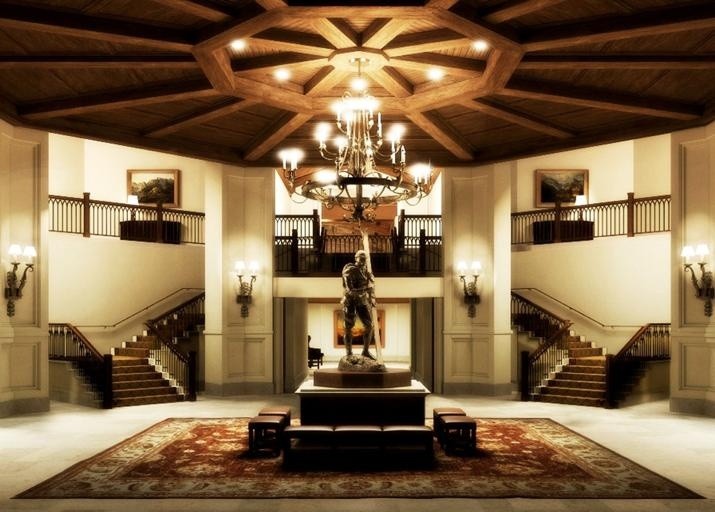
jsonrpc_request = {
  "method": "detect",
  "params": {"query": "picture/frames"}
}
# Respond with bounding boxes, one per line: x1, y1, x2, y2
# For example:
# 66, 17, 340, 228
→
126, 169, 180, 208
535, 168, 590, 210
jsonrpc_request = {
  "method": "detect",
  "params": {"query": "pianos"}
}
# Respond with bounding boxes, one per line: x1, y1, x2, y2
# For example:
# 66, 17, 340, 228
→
308, 335, 324, 369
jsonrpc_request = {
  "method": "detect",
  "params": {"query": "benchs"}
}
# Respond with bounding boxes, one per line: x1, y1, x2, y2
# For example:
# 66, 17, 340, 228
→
281, 424, 433, 471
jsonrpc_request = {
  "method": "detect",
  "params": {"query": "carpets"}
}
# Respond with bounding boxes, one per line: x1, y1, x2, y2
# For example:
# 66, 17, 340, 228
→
9, 417, 708, 499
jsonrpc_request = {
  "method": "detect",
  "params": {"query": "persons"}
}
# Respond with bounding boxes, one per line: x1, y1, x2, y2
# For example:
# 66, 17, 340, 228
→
341, 249, 377, 361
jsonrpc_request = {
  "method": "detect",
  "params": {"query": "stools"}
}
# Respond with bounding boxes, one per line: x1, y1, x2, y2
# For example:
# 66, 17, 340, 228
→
248, 406, 291, 455
432, 407, 477, 453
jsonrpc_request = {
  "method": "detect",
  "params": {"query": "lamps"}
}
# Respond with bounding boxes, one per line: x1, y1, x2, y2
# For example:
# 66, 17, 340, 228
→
276, 61, 439, 220
5, 241, 37, 316
455, 261, 481, 316
681, 244, 714, 317
232, 261, 261, 317
